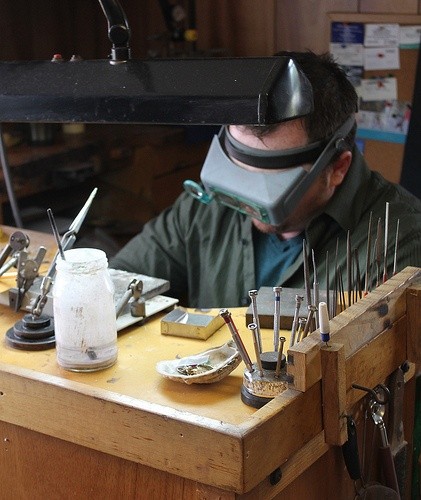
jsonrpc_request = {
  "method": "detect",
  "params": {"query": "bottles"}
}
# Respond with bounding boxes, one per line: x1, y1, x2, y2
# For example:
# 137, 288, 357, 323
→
52, 247, 117, 373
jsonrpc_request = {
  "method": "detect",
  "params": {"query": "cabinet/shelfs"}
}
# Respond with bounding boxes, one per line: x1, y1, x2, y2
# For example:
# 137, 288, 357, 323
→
1, 122, 222, 260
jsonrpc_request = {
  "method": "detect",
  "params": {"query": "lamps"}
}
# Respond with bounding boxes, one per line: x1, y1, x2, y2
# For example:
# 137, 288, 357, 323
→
0, 0, 315, 127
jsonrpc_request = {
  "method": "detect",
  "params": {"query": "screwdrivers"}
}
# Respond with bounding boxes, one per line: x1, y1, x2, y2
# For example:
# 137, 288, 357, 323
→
298, 199, 400, 332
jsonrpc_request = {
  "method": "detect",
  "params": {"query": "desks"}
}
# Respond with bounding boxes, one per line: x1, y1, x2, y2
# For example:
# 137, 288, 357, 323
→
1, 225, 421, 499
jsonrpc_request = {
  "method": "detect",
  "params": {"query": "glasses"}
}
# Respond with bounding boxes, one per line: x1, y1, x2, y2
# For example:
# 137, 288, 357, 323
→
184, 113, 358, 227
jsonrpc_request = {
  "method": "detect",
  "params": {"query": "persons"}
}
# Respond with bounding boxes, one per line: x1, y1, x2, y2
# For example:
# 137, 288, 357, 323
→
103, 47, 420, 312
